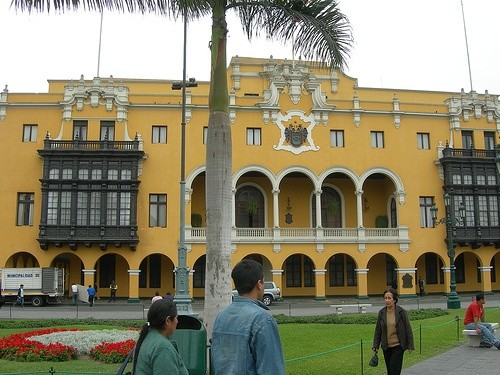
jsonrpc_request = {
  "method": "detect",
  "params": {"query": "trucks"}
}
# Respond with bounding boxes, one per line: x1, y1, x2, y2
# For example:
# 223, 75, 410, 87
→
0, 266, 66, 307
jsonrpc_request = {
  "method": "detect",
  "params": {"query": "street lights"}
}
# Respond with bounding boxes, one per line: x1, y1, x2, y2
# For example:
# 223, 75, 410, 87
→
430, 192, 466, 309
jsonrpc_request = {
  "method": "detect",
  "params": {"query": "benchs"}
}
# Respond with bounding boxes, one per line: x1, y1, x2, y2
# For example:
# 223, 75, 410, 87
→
330, 304, 372, 314
463, 323, 499, 347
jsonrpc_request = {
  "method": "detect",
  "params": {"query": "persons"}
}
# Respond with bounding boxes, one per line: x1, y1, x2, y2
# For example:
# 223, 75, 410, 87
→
372, 288, 415, 375
87, 285, 96, 308
418, 276, 424, 297
391, 279, 397, 289
71, 283, 78, 305
211, 259, 285, 375
133, 299, 189, 375
108, 282, 118, 302
164, 292, 173, 301
464, 293, 500, 351
94, 282, 100, 300
13, 284, 24, 308
151, 291, 162, 304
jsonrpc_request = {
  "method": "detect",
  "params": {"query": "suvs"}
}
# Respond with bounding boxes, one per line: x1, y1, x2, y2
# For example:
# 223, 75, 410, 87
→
232, 282, 281, 306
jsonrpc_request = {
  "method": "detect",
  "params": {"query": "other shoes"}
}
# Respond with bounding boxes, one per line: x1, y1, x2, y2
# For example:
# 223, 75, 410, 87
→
479, 342, 493, 348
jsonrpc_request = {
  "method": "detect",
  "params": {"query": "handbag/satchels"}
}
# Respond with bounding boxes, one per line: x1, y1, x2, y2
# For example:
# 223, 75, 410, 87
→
369, 351, 378, 367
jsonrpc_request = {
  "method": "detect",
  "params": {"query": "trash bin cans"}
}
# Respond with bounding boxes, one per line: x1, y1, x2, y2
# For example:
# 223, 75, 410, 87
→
168, 315, 206, 375
